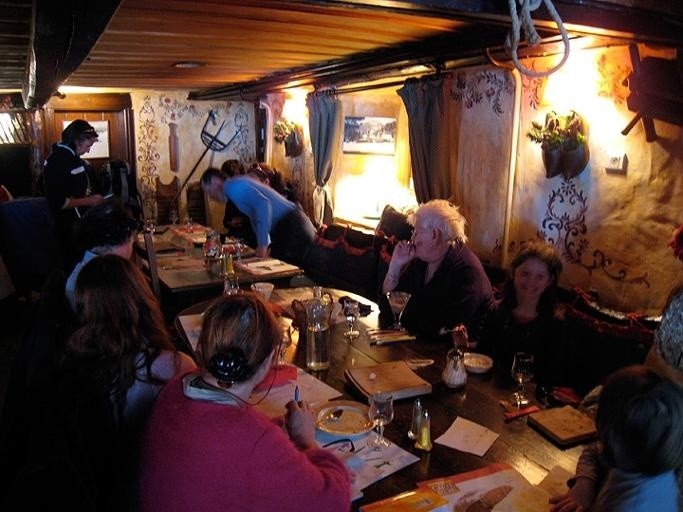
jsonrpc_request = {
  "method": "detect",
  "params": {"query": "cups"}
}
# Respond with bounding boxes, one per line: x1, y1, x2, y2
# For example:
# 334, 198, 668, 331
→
203, 229, 242, 297
303, 300, 332, 372
250, 282, 275, 302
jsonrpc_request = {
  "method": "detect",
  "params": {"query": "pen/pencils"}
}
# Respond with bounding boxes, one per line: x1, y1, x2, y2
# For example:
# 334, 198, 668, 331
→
104, 193, 114, 199
295, 385, 299, 401
365, 329, 416, 345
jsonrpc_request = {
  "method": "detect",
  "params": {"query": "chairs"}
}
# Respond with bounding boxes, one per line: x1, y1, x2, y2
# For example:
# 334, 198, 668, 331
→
133, 233, 161, 299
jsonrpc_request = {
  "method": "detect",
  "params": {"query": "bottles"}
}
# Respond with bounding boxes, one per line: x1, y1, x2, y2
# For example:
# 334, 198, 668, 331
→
415, 453, 430, 482
441, 349, 469, 388
407, 399, 423, 440
312, 286, 323, 299
413, 409, 434, 451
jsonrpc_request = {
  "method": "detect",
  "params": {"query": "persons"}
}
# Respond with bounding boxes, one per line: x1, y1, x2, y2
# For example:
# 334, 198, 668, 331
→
373, 198, 495, 347
544, 362, 682, 512
60, 200, 140, 308
52, 252, 198, 438
198, 158, 331, 287
449, 243, 564, 390
133, 292, 352, 512
42, 119, 104, 232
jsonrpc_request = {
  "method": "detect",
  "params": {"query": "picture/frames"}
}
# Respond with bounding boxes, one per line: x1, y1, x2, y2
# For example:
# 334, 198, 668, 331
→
342, 116, 398, 157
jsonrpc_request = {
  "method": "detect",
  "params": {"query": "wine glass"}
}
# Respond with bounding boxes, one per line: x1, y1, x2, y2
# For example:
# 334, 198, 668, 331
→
511, 351, 535, 405
385, 291, 413, 330
367, 394, 395, 451
169, 210, 178, 229
146, 218, 158, 243
342, 299, 362, 339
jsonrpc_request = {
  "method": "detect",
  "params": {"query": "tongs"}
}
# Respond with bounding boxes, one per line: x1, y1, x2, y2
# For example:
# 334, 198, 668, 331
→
572, 285, 603, 313
625, 311, 664, 334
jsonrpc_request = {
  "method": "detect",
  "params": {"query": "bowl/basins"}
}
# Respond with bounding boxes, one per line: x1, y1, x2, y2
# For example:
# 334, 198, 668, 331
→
637, 316, 664, 332
580, 302, 631, 325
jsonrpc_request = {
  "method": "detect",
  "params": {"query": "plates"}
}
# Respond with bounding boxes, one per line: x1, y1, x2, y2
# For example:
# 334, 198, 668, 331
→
463, 352, 494, 374
313, 400, 377, 437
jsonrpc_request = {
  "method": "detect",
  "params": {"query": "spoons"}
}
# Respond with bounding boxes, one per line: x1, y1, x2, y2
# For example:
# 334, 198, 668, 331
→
438, 326, 457, 335
314, 409, 344, 427
